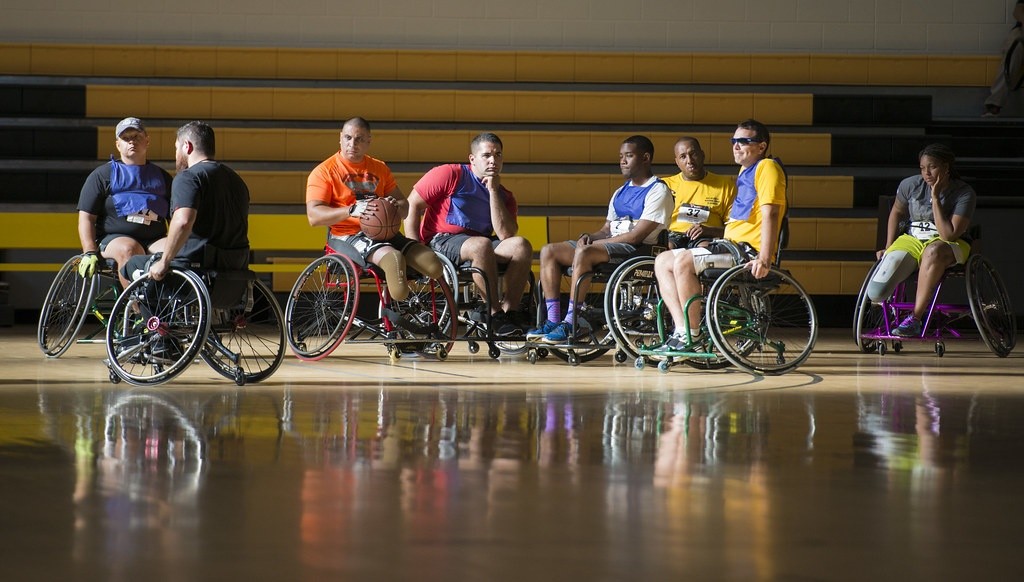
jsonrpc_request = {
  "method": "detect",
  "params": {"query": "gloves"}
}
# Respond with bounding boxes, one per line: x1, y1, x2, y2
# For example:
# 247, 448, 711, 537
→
78, 251, 102, 278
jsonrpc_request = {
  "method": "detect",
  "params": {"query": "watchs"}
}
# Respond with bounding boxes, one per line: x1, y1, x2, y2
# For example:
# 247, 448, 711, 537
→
578, 232, 591, 240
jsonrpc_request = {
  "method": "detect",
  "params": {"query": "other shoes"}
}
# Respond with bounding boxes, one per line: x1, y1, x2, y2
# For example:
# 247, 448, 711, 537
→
132, 317, 149, 333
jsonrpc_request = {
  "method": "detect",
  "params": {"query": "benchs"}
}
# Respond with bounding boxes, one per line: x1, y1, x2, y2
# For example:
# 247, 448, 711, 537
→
0, 212, 549, 272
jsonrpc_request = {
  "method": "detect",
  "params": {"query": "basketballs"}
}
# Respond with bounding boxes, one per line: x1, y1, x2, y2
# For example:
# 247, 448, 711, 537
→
360, 199, 401, 240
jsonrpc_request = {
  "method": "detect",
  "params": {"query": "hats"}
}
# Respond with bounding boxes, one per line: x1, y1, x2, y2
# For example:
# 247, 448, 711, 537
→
115, 117, 146, 138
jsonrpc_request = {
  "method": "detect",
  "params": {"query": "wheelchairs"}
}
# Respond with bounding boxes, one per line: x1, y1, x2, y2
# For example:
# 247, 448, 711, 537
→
851, 216, 1019, 360
283, 220, 459, 363
103, 244, 287, 387
33, 238, 222, 361
395, 213, 820, 377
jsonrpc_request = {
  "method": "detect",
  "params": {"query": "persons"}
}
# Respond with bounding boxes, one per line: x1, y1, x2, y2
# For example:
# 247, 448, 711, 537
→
76, 117, 175, 319
120, 121, 250, 363
658, 136, 737, 253
304, 118, 444, 302
648, 119, 785, 363
875, 143, 977, 337
526, 135, 675, 345
403, 132, 533, 335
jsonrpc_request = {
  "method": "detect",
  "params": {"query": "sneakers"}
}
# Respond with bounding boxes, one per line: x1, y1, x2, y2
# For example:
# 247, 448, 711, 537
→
171, 352, 183, 364
542, 321, 582, 345
493, 316, 535, 336
648, 333, 681, 361
659, 329, 709, 362
526, 319, 558, 341
130, 345, 172, 365
891, 313, 921, 336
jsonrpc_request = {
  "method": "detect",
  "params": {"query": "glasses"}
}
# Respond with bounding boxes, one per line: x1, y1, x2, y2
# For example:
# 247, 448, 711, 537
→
731, 138, 768, 145
